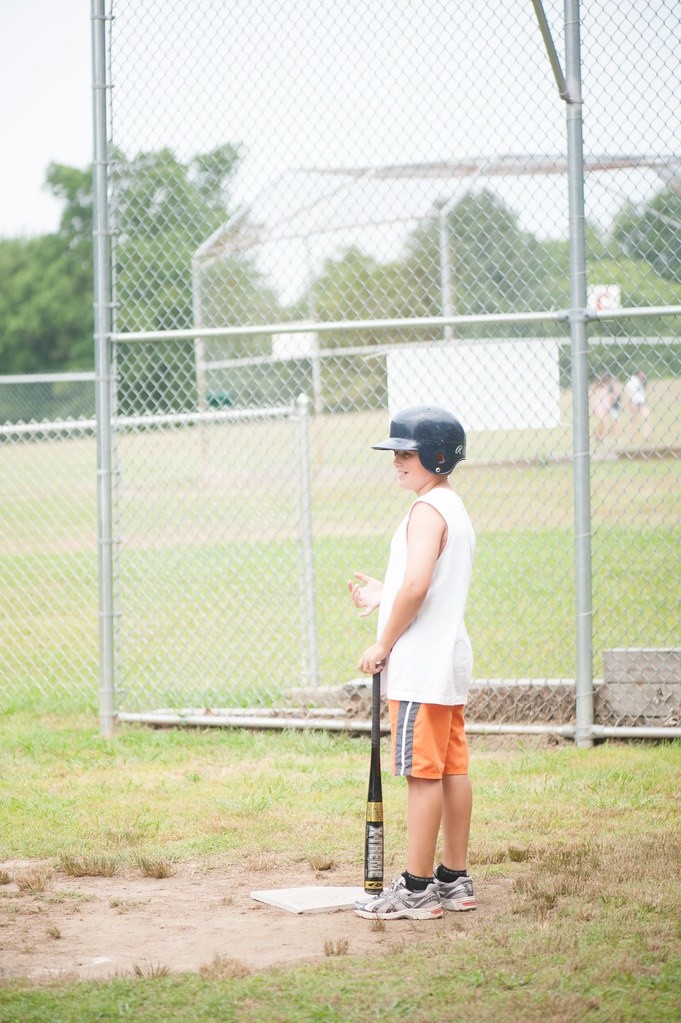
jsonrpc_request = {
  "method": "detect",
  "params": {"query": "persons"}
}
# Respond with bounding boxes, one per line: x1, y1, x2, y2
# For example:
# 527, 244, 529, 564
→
344, 406, 478, 919
591, 368, 661, 448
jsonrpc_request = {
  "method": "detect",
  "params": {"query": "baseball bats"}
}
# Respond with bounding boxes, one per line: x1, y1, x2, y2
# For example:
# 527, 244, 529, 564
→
364, 667, 384, 895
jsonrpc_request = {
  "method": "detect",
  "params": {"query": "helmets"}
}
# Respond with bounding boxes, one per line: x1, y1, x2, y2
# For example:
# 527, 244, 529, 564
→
371, 406, 466, 475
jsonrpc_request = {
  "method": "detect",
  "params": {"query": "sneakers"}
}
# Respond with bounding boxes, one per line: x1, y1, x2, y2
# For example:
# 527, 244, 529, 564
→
433, 871, 476, 912
353, 878, 443, 920
